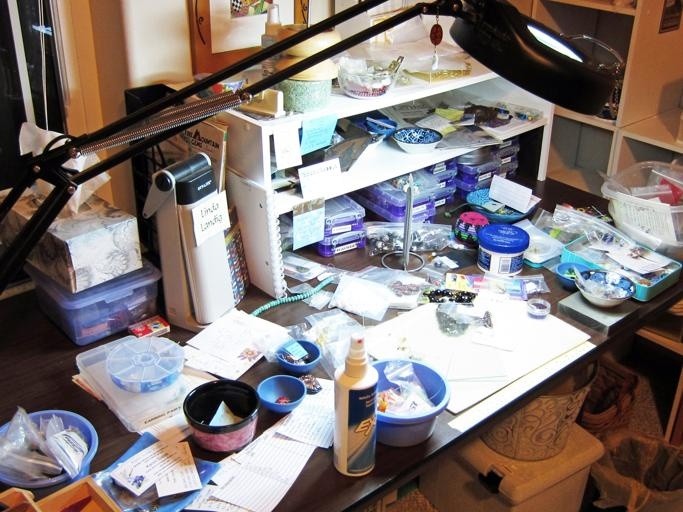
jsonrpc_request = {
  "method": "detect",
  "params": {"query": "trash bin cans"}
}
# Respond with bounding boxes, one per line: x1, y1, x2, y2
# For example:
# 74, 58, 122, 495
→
591, 432, 683, 512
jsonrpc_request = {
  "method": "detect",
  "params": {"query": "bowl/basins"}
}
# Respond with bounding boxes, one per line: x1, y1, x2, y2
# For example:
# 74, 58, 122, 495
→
0, 409, 99, 489
183, 379, 261, 455
369, 357, 451, 447
392, 127, 444, 156
575, 270, 636, 308
366, 119, 398, 143
256, 374, 307, 414
556, 261, 592, 293
275, 339, 322, 373
336, 56, 400, 100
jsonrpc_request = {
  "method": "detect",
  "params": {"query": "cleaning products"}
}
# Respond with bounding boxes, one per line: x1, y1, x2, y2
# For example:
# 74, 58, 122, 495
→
259, 3, 286, 89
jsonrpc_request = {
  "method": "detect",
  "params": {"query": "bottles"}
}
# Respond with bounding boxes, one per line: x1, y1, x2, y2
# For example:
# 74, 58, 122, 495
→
476, 223, 530, 277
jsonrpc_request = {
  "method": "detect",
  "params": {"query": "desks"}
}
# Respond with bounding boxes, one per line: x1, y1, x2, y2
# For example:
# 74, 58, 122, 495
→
0, 176, 683, 512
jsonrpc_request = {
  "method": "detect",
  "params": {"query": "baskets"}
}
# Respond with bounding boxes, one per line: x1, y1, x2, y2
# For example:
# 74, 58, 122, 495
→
582, 355, 641, 439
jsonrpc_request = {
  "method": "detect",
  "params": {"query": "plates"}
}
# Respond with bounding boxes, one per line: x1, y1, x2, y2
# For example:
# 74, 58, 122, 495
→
94, 431, 222, 512
467, 188, 536, 224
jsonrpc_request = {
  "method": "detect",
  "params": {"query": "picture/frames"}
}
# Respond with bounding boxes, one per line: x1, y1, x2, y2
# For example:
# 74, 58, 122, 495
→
185, 0, 309, 80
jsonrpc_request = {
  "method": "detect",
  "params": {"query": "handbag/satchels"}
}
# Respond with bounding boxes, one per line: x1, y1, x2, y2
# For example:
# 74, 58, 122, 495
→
590, 63, 625, 121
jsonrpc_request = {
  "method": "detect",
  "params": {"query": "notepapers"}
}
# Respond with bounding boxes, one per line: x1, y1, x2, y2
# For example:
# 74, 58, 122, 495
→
434, 108, 464, 122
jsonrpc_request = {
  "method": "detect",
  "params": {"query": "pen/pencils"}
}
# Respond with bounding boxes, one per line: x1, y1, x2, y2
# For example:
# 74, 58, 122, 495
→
489, 107, 532, 122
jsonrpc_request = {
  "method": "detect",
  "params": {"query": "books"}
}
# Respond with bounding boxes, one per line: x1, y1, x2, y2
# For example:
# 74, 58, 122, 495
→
145, 114, 252, 308
432, 125, 503, 152
466, 100, 542, 136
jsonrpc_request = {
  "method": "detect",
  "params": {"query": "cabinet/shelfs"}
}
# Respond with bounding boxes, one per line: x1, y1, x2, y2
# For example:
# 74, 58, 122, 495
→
164, 52, 554, 298
513, 1, 682, 200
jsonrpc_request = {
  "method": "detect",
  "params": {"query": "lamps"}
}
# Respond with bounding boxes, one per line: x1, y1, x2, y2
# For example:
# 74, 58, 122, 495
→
1, 0, 614, 298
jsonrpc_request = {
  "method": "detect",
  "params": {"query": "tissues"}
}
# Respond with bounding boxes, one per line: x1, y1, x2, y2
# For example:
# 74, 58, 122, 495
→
0, 122, 145, 295
